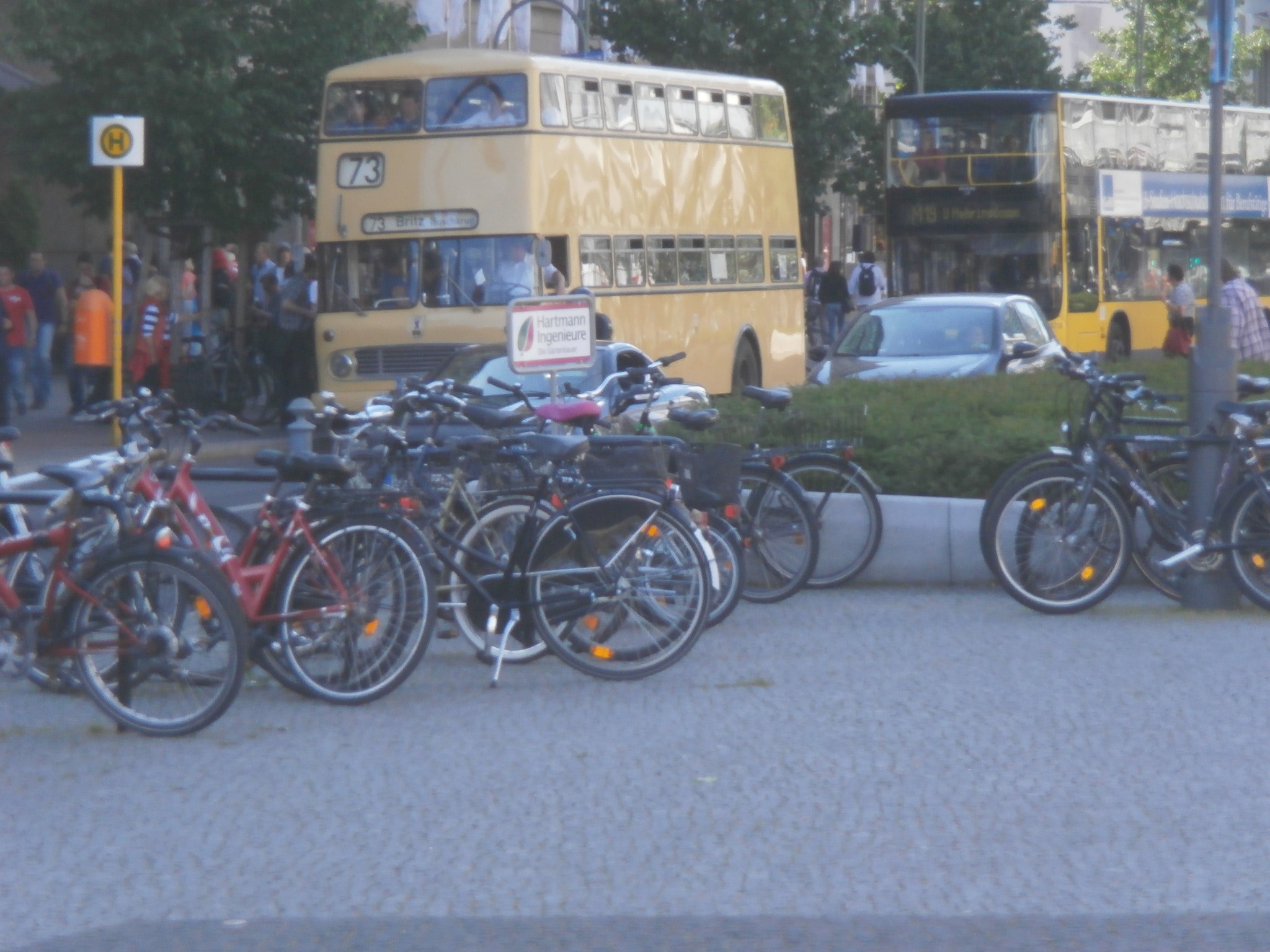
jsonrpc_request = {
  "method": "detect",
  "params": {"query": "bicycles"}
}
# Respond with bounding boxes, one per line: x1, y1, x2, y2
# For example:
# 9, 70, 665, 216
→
976, 338, 1270, 616
1, 323, 884, 735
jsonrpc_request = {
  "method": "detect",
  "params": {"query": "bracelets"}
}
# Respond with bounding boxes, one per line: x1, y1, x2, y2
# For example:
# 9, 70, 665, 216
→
1165, 300, 1169, 303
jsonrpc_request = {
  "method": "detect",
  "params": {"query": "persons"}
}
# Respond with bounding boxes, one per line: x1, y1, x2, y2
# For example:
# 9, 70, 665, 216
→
1220, 256, 1270, 364
847, 250, 888, 311
330, 82, 517, 129
818, 261, 855, 346
0, 242, 476, 432
913, 130, 1036, 183
494, 241, 566, 296
1158, 264, 1196, 358
804, 258, 826, 346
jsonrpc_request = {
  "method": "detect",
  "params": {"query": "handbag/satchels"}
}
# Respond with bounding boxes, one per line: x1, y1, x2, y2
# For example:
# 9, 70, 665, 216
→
1163, 308, 1191, 357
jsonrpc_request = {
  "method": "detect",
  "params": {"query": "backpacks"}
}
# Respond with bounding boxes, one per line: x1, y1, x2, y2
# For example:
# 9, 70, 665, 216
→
859, 263, 876, 297
808, 267, 823, 300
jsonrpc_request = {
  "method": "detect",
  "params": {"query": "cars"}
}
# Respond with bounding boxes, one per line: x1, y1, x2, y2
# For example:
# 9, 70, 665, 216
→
801, 293, 1073, 390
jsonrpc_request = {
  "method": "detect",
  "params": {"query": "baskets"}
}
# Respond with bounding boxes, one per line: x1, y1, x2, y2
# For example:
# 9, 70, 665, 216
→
675, 439, 743, 511
782, 404, 867, 451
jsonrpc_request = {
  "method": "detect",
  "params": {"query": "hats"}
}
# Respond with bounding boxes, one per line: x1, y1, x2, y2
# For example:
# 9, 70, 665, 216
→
215, 248, 227, 271
79, 249, 96, 261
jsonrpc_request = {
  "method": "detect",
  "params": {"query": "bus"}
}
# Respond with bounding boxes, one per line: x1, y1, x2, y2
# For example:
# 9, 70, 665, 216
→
851, 89, 1270, 355
312, 47, 805, 423
